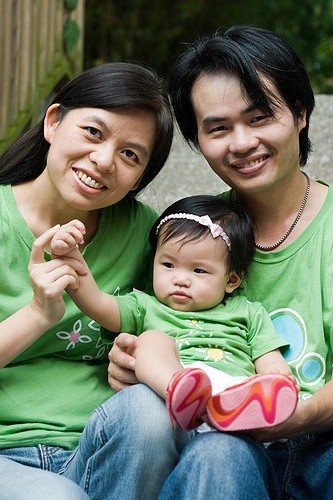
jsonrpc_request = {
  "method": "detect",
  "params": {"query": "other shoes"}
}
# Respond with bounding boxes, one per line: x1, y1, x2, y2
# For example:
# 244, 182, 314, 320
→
206, 374, 299, 432
165, 368, 213, 433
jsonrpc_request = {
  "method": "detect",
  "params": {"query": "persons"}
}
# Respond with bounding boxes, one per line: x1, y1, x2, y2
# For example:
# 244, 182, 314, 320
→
50, 194, 300, 432
0, 62, 195, 499
105, 25, 333, 500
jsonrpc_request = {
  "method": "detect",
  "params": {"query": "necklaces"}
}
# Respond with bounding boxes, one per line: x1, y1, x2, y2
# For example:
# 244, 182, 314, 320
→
228, 170, 309, 251
